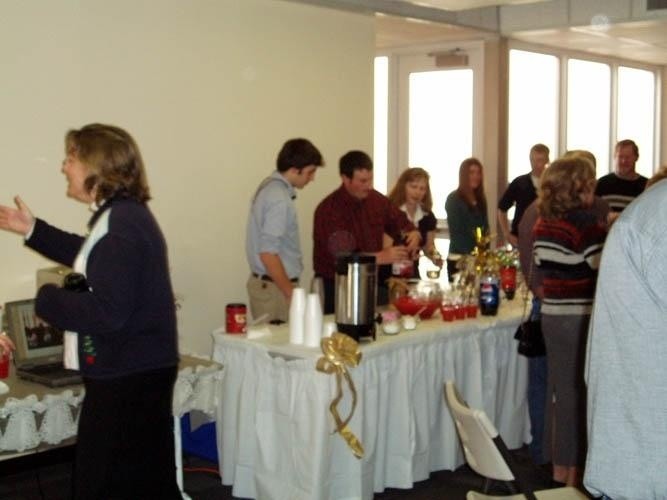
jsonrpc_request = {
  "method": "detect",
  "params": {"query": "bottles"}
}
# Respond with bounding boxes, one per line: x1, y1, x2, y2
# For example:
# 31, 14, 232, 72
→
499, 249, 516, 300
399, 235, 414, 278
401, 315, 416, 330
479, 269, 499, 316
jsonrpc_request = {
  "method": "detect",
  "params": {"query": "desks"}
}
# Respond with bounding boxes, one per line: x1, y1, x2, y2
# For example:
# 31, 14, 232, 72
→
213, 292, 532, 499
0, 351, 224, 500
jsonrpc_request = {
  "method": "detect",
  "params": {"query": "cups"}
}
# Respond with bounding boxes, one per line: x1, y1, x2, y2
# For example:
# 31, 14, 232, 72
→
63, 274, 86, 293
426, 256, 440, 279
440, 289, 478, 322
0, 348, 9, 380
287, 287, 336, 349
382, 316, 400, 335
224, 304, 247, 335
310, 276, 325, 306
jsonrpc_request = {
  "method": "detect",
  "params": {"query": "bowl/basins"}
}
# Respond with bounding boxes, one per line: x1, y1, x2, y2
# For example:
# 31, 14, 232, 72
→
392, 296, 442, 319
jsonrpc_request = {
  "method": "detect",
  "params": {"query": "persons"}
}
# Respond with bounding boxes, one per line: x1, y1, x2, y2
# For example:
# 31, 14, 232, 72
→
243, 136, 326, 327
597, 138, 649, 227
582, 174, 665, 500
513, 148, 611, 474
0, 120, 188, 500
0, 331, 18, 361
495, 142, 550, 251
311, 149, 423, 314
522, 157, 607, 489
444, 156, 490, 283
377, 167, 444, 307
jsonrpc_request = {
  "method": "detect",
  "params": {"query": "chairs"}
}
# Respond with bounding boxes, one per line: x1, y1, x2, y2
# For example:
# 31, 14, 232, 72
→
439, 376, 590, 500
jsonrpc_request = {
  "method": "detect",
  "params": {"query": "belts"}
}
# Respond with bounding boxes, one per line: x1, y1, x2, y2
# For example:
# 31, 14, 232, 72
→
253, 273, 299, 282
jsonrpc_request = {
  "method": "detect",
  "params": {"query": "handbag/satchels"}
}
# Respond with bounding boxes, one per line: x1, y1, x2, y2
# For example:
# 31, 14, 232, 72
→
513, 320, 546, 359
